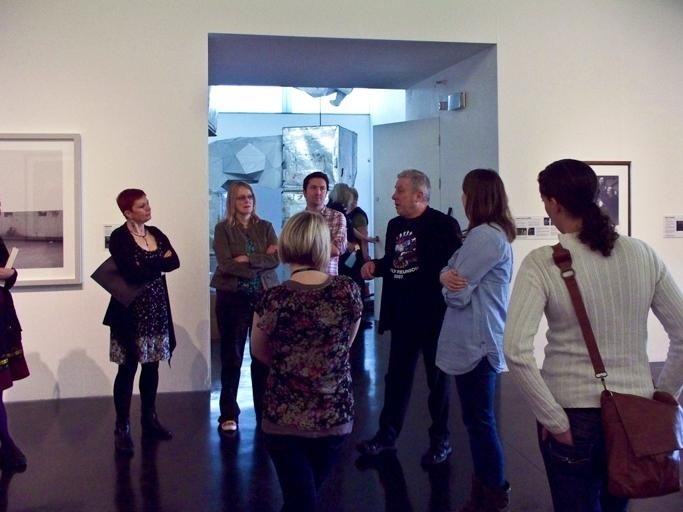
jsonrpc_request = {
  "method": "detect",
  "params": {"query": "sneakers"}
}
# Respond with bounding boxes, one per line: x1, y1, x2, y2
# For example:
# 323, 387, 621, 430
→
114, 421, 133, 452
421, 440, 453, 466
453, 479, 511, 512
350, 367, 370, 384
0, 440, 27, 466
221, 420, 238, 431
142, 420, 172, 440
356, 431, 398, 456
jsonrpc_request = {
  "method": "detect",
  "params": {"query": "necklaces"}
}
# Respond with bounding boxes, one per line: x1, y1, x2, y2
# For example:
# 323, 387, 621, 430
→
129, 229, 150, 247
289, 266, 318, 277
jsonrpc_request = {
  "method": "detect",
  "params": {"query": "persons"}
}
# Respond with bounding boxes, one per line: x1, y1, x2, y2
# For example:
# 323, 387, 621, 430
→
210, 181, 281, 431
435, 169, 516, 512
0, 235, 30, 473
326, 183, 372, 329
354, 169, 463, 464
302, 171, 348, 276
346, 187, 372, 281
504, 159, 683, 511
250, 212, 364, 510
103, 189, 180, 449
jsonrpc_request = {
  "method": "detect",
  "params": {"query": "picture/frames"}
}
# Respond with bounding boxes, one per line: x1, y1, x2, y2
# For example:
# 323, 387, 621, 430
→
582, 161, 631, 239
0, 133, 82, 289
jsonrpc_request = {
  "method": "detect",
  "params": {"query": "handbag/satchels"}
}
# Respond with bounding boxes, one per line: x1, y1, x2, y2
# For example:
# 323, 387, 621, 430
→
600, 390, 683, 498
